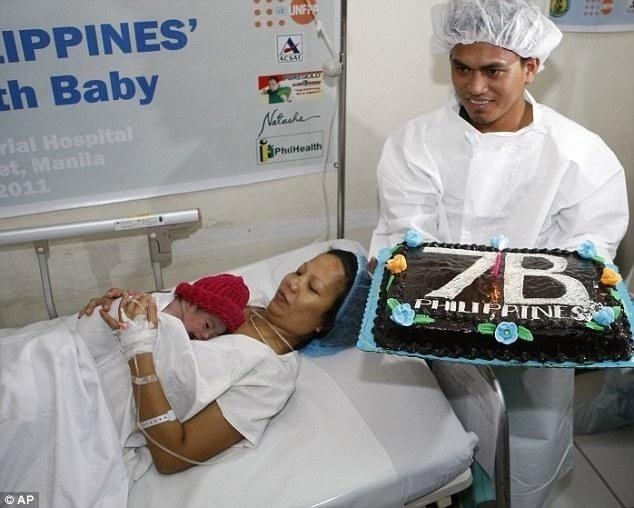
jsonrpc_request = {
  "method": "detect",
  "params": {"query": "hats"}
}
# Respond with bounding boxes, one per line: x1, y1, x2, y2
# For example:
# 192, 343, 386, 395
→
175, 274, 250, 334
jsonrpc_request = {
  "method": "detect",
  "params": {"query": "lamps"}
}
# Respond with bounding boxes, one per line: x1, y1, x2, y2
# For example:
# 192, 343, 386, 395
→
322, 56, 343, 77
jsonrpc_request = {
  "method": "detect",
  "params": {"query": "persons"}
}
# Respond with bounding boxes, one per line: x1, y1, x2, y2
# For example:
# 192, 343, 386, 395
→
77, 246, 372, 508
160, 271, 250, 340
260, 77, 291, 105
363, 0, 632, 508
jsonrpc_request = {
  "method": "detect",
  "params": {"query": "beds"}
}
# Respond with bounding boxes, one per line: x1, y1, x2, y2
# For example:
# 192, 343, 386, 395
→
0, 208, 484, 507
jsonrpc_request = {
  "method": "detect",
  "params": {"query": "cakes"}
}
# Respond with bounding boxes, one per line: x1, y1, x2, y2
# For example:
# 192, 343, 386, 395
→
371, 229, 634, 364
